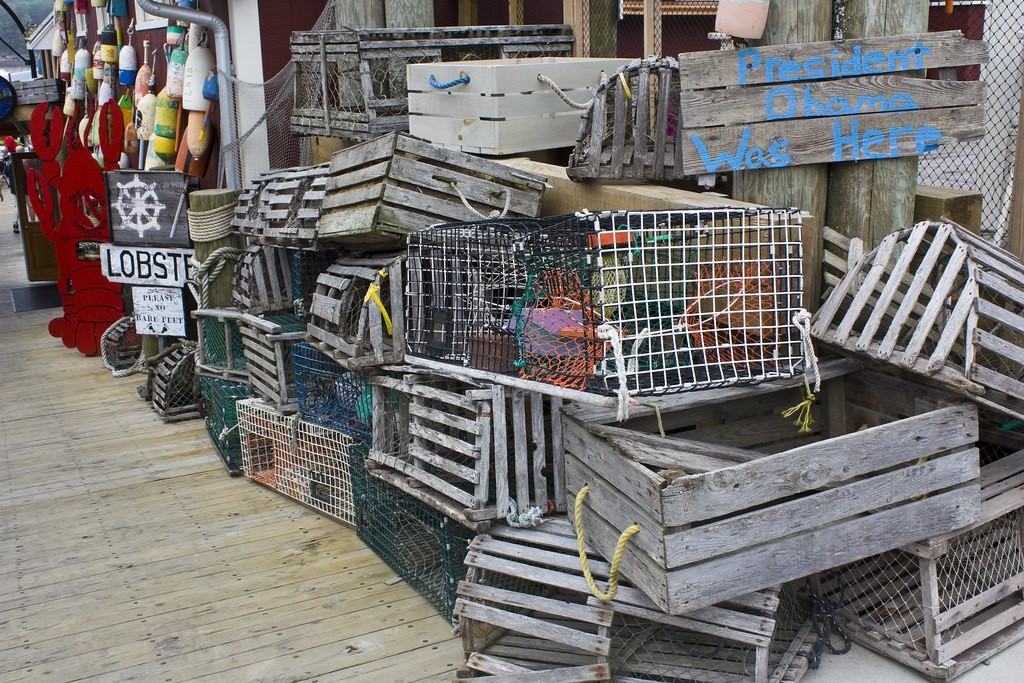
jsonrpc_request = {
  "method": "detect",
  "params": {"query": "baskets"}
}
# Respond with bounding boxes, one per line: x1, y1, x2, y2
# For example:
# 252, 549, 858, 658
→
152, 161, 816, 622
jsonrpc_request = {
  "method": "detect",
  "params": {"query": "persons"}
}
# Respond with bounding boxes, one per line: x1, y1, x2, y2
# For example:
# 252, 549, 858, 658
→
0, 145, 35, 233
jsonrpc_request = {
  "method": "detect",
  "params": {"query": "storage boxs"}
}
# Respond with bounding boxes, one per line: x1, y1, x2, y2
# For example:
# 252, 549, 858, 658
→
316, 135, 549, 245
11, 78, 62, 103
407, 58, 640, 156
362, 372, 568, 529
558, 370, 981, 615
